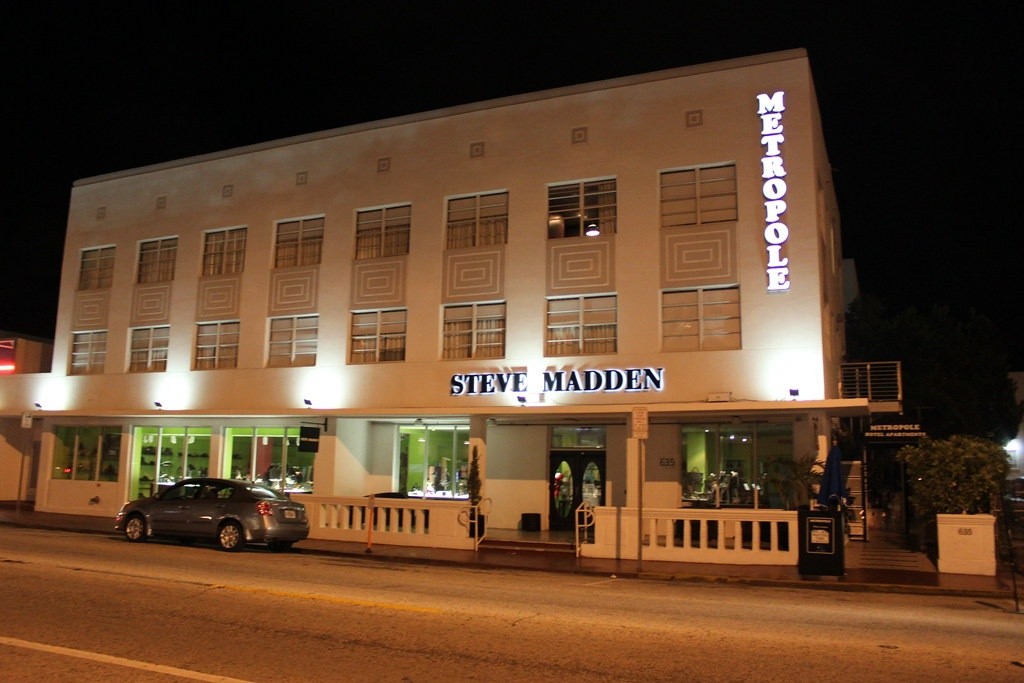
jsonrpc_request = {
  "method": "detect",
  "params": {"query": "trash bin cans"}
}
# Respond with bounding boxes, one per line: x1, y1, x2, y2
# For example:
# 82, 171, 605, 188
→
797, 505, 847, 582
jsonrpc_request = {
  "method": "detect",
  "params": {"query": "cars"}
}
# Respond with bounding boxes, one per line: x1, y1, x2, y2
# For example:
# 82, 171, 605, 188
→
114, 477, 310, 553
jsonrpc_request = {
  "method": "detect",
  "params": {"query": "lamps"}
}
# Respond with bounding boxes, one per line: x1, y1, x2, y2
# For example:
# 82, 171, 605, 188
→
303, 400, 312, 409
143, 435, 146, 443
170, 437, 176, 443
517, 396, 526, 406
188, 436, 195, 444
148, 436, 153, 442
413, 418, 423, 425
155, 401, 163, 409
585, 213, 600, 236
32, 403, 42, 410
262, 437, 268, 445
790, 389, 800, 401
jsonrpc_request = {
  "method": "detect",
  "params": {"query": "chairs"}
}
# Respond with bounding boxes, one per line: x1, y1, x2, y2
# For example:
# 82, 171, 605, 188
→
192, 487, 201, 497
206, 489, 218, 497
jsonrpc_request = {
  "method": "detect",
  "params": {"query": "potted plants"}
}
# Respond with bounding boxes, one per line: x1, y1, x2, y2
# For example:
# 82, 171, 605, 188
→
464, 443, 487, 537
893, 432, 1011, 577
